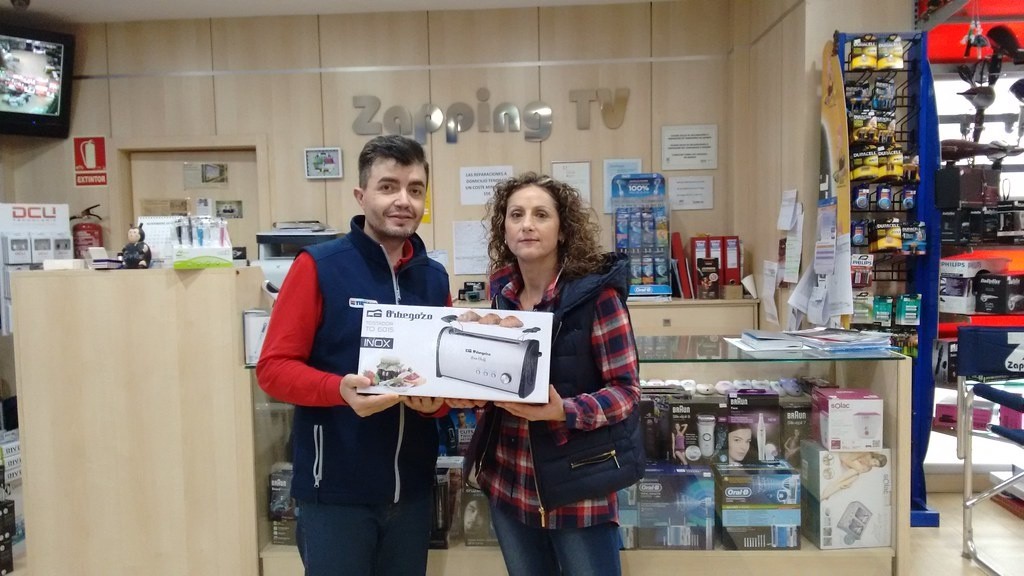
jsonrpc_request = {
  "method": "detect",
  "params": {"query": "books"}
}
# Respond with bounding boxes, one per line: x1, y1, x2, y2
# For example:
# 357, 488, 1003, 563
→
741, 329, 803, 352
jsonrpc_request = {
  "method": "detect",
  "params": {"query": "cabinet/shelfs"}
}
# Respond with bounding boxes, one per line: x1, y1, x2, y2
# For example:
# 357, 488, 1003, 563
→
245, 336, 913, 576
626, 299, 760, 336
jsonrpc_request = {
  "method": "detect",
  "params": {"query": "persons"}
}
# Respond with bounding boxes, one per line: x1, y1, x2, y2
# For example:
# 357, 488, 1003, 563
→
255, 136, 452, 576
728, 423, 753, 464
837, 451, 888, 489
463, 497, 490, 541
784, 426, 802, 468
450, 412, 476, 443
671, 422, 689, 466
446, 171, 644, 576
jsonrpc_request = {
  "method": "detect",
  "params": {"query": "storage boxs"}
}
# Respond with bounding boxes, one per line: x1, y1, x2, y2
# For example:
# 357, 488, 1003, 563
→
617, 378, 892, 552
268, 470, 298, 546
428, 406, 500, 550
356, 302, 555, 404
0, 441, 23, 576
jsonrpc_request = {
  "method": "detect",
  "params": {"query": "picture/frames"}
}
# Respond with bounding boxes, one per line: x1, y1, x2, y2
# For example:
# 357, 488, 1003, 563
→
304, 148, 343, 179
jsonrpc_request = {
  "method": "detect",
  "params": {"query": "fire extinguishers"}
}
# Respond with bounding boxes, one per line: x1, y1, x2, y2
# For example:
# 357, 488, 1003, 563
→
70, 204, 104, 259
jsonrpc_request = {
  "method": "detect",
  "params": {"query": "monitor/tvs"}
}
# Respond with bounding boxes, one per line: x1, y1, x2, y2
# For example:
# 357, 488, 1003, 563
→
0, 24, 76, 140
